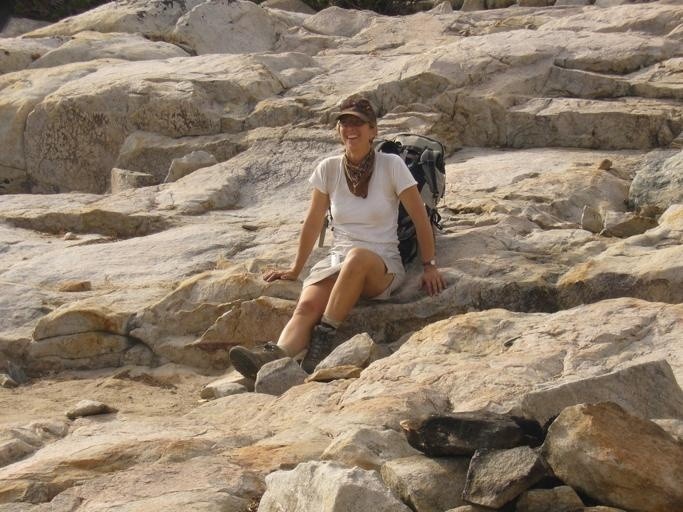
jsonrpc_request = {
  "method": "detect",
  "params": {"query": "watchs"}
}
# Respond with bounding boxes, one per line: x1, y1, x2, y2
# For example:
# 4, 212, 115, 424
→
422, 259, 437, 266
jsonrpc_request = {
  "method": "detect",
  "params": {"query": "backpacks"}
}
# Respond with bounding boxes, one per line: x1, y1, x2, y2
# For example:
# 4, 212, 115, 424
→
375, 132, 446, 263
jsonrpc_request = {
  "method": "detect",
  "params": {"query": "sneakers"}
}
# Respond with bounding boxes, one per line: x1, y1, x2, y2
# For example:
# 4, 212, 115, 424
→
229, 341, 289, 381
300, 325, 337, 374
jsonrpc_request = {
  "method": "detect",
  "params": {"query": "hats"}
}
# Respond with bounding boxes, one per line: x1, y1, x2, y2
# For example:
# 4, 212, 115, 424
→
333, 97, 376, 128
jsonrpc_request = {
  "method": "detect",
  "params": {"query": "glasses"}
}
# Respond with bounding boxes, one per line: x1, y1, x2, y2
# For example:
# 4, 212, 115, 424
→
338, 117, 368, 127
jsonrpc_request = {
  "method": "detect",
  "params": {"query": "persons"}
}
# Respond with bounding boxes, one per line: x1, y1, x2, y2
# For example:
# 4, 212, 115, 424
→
228, 96, 448, 384
